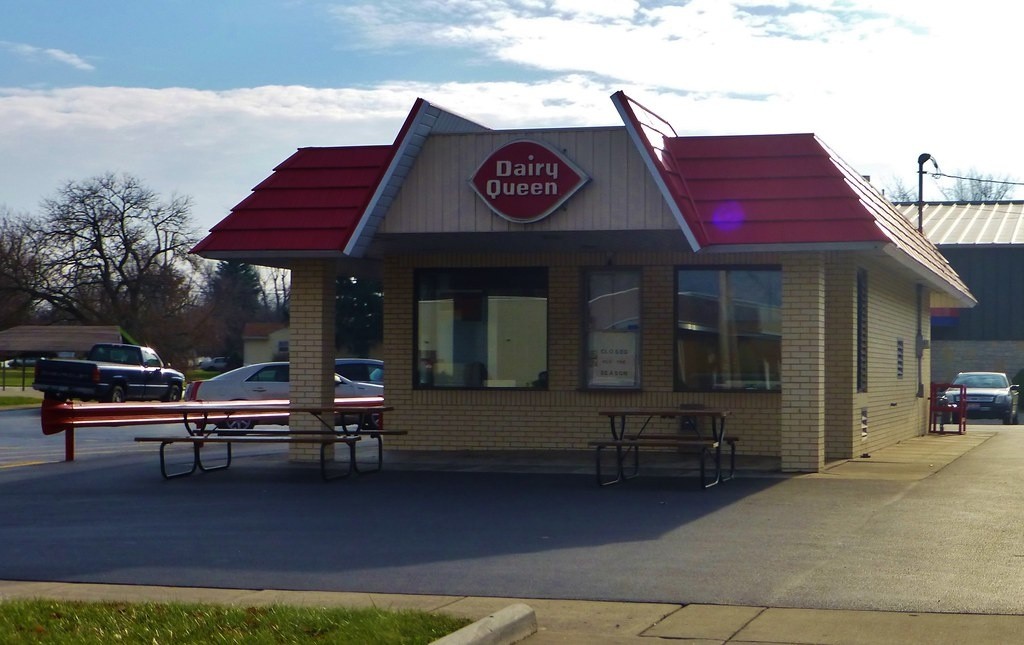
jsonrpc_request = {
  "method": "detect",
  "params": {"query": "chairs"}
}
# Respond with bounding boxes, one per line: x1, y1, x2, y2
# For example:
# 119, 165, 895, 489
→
928, 382, 966, 434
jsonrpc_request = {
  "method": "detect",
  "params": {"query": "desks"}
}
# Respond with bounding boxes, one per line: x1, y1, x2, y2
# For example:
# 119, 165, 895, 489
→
600, 408, 731, 483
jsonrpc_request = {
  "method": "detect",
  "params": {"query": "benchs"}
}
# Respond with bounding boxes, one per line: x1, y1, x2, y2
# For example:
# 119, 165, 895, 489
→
587, 440, 719, 489
194, 430, 408, 475
134, 436, 361, 481
624, 434, 740, 481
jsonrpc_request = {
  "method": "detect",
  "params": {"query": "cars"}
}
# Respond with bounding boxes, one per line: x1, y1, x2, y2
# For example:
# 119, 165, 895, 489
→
6, 350, 57, 369
203, 356, 230, 370
940, 370, 1021, 423
180, 360, 383, 436
328, 357, 384, 385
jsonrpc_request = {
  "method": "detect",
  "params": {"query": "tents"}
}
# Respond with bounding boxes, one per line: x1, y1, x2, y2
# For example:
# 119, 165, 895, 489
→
0, 326, 141, 390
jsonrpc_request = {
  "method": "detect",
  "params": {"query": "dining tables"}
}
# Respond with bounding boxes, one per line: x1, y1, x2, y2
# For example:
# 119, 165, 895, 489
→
154, 406, 394, 474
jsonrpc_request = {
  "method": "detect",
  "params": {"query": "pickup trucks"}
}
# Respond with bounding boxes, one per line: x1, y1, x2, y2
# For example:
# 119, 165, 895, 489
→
33, 341, 184, 407
